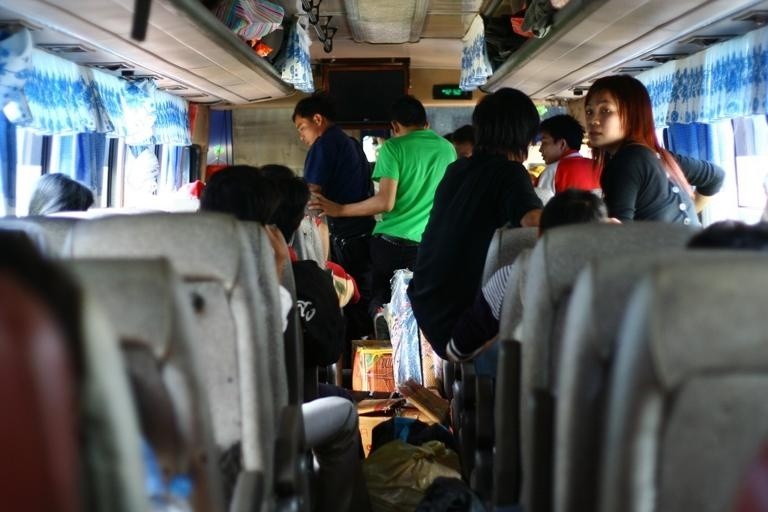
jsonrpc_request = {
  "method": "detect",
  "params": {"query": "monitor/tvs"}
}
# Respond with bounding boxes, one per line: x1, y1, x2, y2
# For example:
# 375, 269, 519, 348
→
321, 57, 411, 127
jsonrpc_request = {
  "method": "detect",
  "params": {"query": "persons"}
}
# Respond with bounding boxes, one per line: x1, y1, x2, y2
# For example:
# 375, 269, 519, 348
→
308, 92, 459, 326
434, 74, 766, 398
292, 92, 374, 278
28, 153, 367, 511
